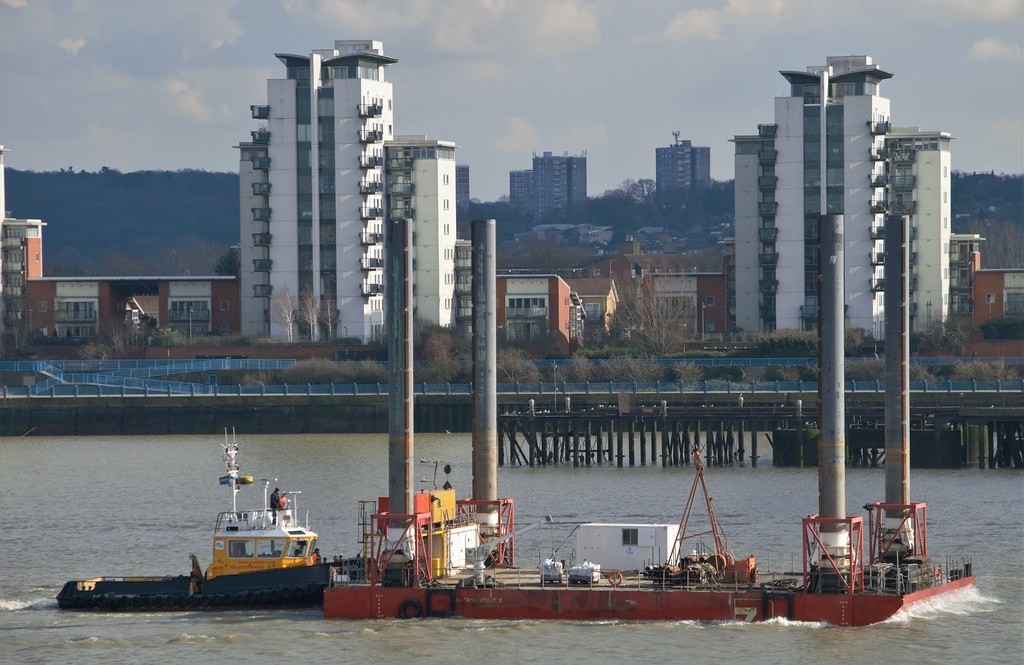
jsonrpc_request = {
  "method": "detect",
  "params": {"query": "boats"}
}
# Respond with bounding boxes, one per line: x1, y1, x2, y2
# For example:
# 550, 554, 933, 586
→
53, 423, 375, 615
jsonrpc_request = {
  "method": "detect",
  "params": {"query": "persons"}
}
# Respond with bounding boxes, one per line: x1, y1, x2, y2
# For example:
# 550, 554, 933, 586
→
311, 548, 321, 564
270, 488, 287, 524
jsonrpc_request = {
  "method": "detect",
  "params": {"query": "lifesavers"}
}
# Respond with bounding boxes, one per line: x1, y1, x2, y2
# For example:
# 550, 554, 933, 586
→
73, 581, 328, 609
934, 566, 944, 580
609, 571, 622, 585
398, 597, 422, 619
709, 554, 727, 571
